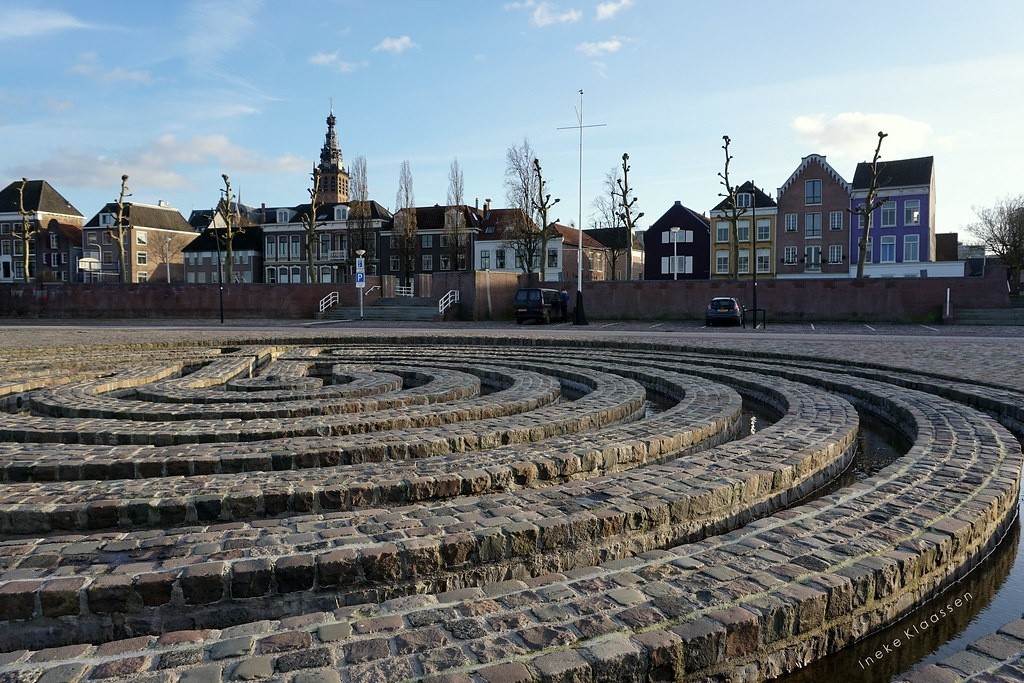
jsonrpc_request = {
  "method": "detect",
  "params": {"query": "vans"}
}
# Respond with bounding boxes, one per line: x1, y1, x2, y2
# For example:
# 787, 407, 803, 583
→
514, 289, 564, 324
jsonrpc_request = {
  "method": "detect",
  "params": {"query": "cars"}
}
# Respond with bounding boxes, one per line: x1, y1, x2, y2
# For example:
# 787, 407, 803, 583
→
706, 298, 745, 326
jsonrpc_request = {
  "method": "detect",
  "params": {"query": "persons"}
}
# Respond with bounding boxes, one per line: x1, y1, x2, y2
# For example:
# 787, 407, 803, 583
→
560, 288, 569, 324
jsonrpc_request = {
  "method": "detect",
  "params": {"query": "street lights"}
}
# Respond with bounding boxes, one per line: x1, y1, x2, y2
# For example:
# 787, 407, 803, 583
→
747, 186, 757, 329
201, 214, 224, 324
